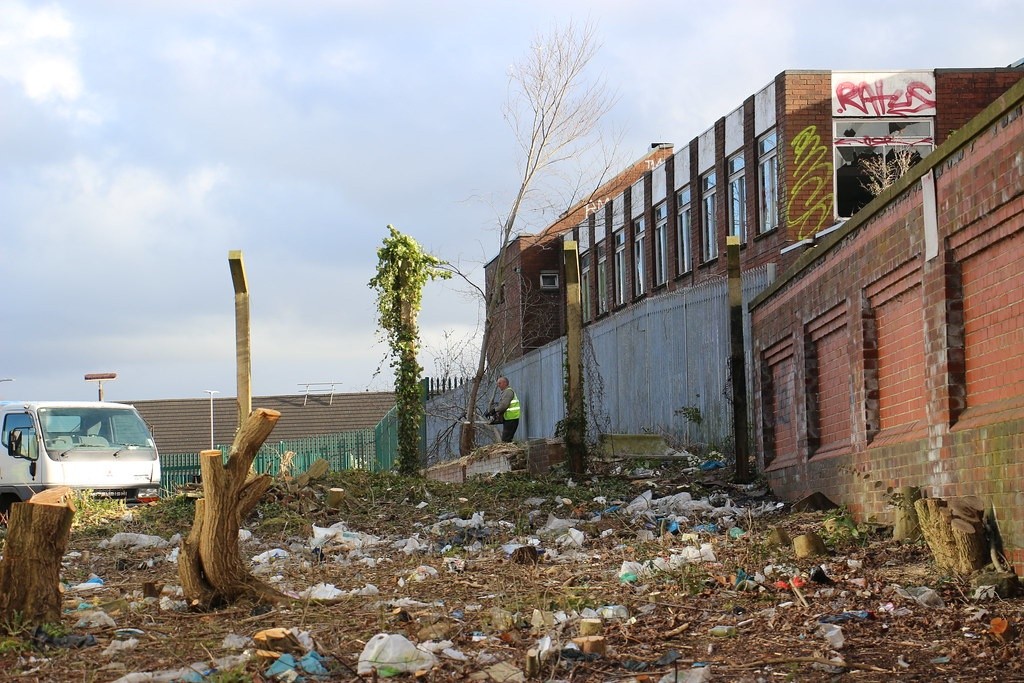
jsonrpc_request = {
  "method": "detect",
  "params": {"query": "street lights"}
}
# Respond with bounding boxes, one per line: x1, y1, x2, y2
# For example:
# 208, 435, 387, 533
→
203, 390, 220, 449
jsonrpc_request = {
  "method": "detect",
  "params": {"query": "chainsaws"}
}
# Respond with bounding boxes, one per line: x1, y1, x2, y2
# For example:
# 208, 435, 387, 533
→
464, 409, 503, 425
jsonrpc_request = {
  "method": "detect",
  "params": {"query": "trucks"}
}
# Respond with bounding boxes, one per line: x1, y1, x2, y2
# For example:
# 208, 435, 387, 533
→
0, 400, 161, 509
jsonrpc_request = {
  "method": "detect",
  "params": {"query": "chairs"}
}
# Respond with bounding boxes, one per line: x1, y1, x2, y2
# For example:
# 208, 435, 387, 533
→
79, 416, 110, 446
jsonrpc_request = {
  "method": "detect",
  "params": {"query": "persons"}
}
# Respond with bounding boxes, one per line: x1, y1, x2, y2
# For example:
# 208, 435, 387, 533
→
484, 376, 520, 445
